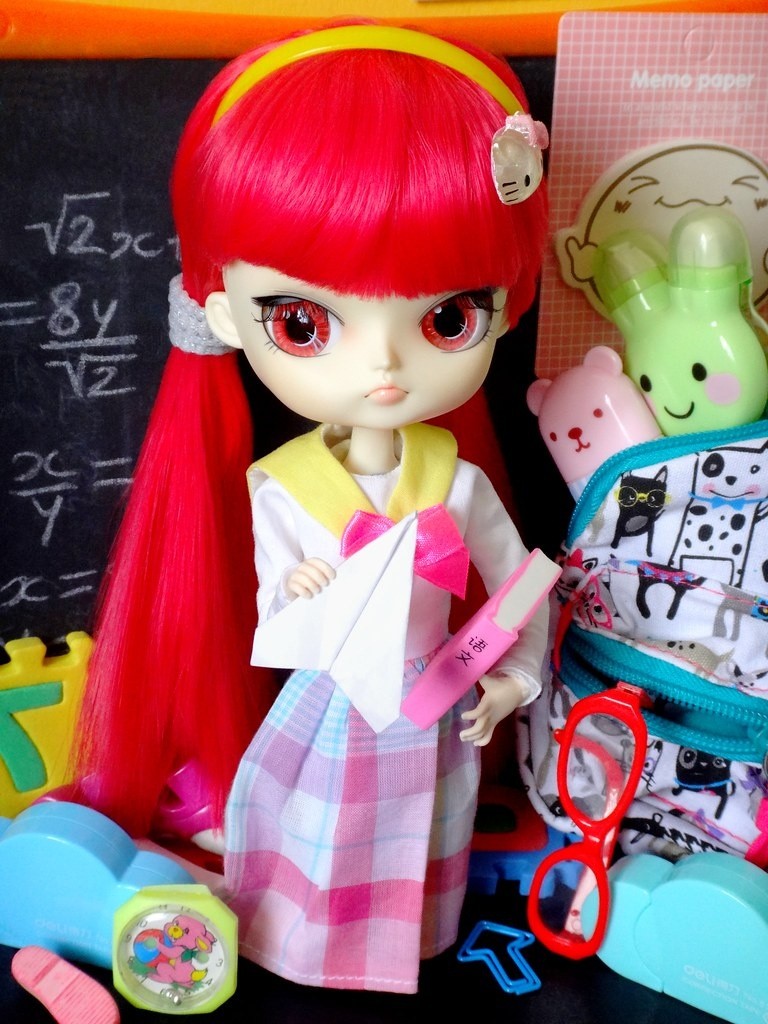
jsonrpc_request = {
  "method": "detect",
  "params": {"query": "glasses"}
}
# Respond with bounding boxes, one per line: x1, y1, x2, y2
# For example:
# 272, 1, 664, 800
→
528, 678, 648, 961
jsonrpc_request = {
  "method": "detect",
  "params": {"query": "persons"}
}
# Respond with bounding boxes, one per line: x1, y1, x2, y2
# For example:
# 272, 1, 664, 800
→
63, 17, 555, 998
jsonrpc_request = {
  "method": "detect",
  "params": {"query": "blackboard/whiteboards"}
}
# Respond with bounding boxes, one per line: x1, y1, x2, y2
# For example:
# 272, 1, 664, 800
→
0, 50, 561, 664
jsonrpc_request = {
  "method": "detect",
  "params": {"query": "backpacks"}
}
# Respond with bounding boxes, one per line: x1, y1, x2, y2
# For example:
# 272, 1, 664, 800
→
521, 420, 767, 871
